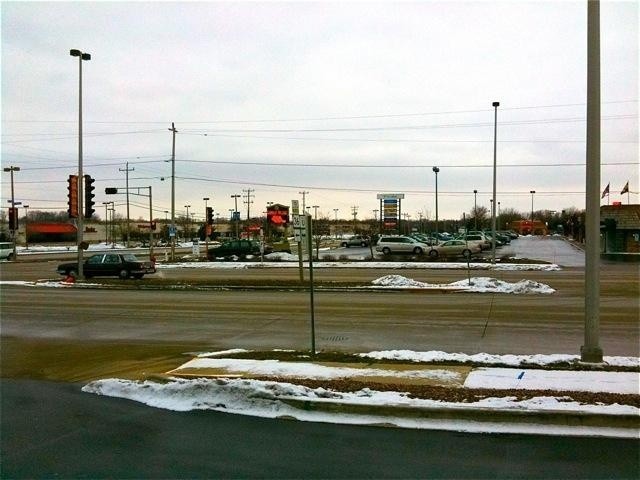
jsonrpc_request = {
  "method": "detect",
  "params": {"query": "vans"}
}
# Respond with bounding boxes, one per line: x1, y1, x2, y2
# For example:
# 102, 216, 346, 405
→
0, 241, 19, 261
206, 238, 262, 262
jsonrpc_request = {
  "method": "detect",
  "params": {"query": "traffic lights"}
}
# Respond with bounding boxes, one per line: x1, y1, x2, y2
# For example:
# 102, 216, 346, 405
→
67, 175, 79, 219
83, 173, 96, 219
8, 207, 15, 230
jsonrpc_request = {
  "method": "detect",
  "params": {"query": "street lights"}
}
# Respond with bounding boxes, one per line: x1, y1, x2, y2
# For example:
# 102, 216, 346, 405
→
491, 101, 499, 265
103, 201, 118, 248
70, 49, 93, 282
530, 190, 536, 234
490, 199, 501, 231
432, 166, 439, 245
23, 204, 30, 250
473, 189, 478, 233
162, 187, 380, 245
4, 165, 21, 261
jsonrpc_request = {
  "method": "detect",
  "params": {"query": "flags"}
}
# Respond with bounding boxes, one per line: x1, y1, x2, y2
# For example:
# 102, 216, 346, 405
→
621, 182, 628, 195
602, 183, 609, 199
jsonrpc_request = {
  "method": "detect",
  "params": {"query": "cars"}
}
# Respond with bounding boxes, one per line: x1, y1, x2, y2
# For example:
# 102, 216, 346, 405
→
340, 230, 520, 260
55, 251, 157, 280
